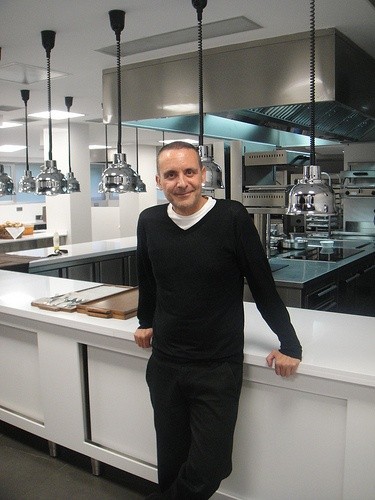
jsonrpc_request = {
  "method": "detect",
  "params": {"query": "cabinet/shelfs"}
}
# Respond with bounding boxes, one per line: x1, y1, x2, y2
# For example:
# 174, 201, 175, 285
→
306, 275, 337, 313
337, 263, 375, 315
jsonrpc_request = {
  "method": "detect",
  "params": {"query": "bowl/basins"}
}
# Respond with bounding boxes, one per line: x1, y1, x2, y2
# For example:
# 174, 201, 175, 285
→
320, 240, 334, 248
280, 239, 309, 249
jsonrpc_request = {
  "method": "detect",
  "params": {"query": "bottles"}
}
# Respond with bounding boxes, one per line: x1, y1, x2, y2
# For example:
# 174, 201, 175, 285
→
53, 232, 60, 250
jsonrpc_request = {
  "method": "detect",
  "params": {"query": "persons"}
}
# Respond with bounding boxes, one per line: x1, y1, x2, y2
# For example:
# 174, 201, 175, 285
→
133, 141, 302, 500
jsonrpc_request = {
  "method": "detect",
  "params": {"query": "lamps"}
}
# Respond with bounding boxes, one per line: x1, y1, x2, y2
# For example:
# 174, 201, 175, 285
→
97, 9, 166, 192
191, 0, 224, 191
286, 0, 339, 216
0, 30, 77, 196
